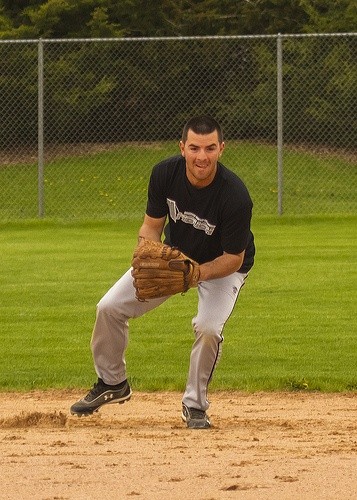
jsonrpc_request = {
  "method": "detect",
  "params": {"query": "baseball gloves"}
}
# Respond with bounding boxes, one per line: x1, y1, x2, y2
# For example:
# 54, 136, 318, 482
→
131, 236, 201, 304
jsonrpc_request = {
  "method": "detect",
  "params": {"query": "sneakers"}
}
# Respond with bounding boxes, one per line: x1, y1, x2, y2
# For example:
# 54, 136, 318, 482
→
182, 401, 212, 428
67, 376, 134, 418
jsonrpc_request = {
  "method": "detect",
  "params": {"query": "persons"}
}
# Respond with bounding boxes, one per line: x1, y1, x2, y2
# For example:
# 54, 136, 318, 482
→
70, 116, 256, 429
